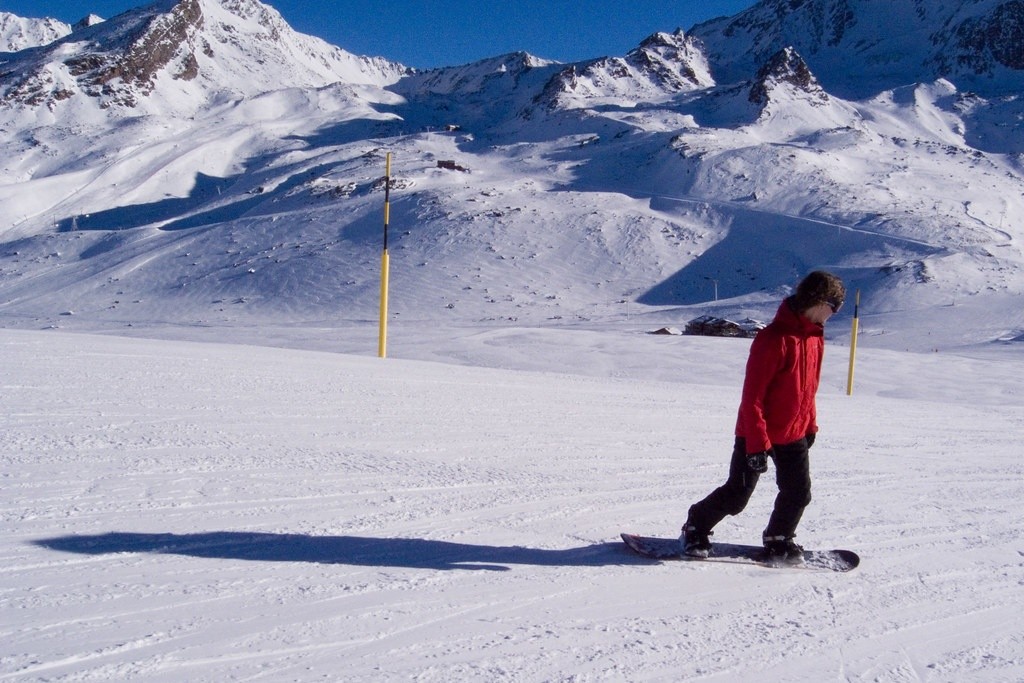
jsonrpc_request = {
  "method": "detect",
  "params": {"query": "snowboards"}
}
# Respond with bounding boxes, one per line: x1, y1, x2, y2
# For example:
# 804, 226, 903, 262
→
620, 533, 860, 574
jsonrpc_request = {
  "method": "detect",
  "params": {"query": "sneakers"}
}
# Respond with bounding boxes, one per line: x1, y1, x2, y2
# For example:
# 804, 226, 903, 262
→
678, 503, 711, 558
763, 521, 802, 560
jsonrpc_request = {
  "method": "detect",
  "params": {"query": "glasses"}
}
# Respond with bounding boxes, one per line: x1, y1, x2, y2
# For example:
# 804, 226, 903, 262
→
832, 301, 844, 314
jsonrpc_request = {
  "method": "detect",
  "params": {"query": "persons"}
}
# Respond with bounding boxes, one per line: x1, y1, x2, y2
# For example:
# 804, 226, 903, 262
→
677, 271, 847, 564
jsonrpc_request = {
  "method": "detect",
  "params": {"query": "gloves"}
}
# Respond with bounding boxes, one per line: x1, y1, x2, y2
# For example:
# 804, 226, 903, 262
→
805, 433, 815, 449
746, 447, 775, 474
